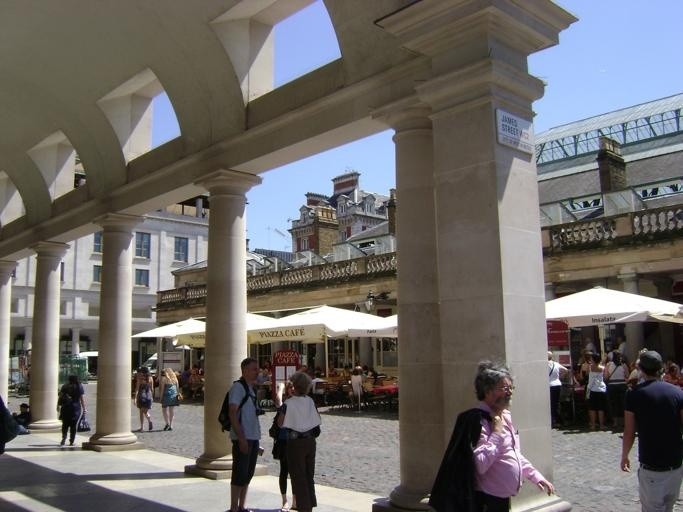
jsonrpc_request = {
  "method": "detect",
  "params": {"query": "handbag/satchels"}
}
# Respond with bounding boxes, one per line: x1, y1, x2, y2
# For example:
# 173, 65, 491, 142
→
585, 385, 591, 400
58, 393, 73, 406
177, 392, 183, 401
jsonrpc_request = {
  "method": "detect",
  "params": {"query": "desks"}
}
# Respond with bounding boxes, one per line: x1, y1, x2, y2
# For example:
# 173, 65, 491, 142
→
191, 366, 586, 422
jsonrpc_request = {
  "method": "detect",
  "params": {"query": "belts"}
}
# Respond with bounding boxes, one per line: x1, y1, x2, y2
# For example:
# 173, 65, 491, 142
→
640, 463, 683, 472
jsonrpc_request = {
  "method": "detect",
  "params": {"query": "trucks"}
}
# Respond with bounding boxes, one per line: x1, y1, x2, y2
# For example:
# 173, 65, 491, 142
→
134, 351, 183, 377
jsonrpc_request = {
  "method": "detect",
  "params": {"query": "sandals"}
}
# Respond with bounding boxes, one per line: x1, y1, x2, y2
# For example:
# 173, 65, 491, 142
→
19, 429, 30, 435
138, 420, 172, 433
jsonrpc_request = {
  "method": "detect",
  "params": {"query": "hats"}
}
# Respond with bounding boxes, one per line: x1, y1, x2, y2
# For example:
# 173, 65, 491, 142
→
20, 403, 31, 408
638, 350, 664, 371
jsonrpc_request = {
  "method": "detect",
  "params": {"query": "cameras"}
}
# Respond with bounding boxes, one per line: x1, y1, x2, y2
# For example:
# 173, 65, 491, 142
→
256, 409, 265, 415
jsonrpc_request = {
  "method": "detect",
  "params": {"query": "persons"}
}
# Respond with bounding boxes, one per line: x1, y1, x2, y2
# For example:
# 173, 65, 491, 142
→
57, 375, 87, 446
269, 381, 297, 512
11, 403, 30, 427
547, 351, 579, 429
228, 358, 261, 512
132, 363, 204, 400
458, 360, 555, 512
276, 372, 322, 512
574, 341, 683, 512
134, 366, 154, 432
256, 361, 378, 410
159, 368, 179, 431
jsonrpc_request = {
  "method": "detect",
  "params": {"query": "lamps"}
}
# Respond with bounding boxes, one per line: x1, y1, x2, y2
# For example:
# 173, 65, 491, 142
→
173, 337, 179, 347
178, 280, 190, 309
364, 289, 392, 312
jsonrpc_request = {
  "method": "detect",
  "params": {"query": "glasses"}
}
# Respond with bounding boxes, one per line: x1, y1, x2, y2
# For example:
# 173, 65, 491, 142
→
497, 385, 516, 394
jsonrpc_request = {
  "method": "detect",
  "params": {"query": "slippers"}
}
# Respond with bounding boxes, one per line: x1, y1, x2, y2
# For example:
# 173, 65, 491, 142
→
292, 503, 297, 510
281, 506, 289, 512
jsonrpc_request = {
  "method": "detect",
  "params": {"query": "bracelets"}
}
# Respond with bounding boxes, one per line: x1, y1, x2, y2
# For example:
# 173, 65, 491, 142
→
83, 412, 87, 414
83, 410, 87, 412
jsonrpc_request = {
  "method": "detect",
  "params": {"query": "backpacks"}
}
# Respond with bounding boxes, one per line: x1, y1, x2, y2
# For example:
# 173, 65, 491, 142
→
218, 379, 250, 432
